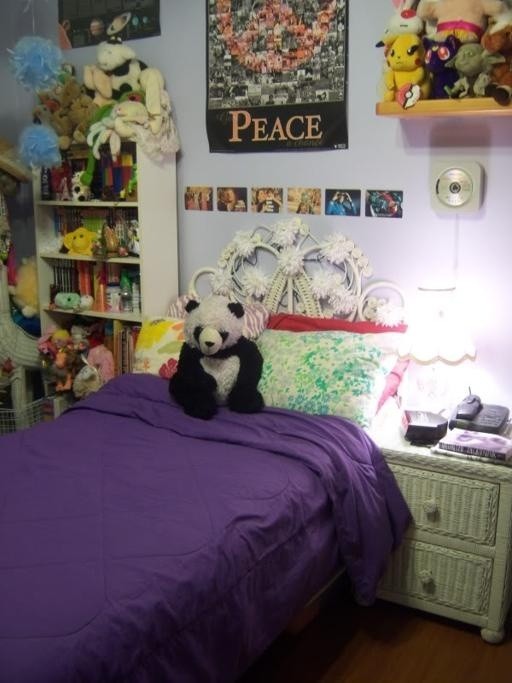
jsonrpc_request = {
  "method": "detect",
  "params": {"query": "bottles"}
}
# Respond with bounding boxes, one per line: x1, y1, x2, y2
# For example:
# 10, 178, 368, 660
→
97, 283, 107, 310
119, 269, 131, 290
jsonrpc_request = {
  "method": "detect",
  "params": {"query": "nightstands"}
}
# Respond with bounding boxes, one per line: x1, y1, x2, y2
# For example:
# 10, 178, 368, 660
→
376, 434, 512, 644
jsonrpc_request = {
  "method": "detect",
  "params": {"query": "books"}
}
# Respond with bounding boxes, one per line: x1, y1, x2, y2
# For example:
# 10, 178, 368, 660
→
42, 159, 140, 377
431, 442, 505, 464
438, 426, 512, 459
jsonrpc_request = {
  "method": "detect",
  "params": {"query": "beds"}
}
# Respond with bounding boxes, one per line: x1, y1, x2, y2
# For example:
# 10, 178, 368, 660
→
0, 217, 407, 671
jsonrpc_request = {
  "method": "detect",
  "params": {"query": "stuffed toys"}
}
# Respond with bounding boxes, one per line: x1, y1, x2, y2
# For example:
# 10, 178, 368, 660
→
4, 30, 182, 172
169, 296, 266, 420
38, 322, 115, 400
7, 253, 39, 318
369, 0, 511, 105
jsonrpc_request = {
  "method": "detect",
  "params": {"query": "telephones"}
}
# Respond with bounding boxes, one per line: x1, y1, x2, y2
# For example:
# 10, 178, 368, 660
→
450, 394, 509, 435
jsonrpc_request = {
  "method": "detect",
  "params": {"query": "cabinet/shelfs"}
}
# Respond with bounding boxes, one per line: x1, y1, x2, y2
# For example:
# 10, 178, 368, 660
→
32, 134, 182, 323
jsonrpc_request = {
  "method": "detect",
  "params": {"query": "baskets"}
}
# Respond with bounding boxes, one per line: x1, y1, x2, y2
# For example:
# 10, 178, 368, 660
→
0, 398, 42, 436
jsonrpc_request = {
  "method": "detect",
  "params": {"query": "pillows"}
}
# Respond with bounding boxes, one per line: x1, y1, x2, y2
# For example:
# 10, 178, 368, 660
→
131, 316, 184, 376
266, 314, 409, 413
252, 329, 398, 426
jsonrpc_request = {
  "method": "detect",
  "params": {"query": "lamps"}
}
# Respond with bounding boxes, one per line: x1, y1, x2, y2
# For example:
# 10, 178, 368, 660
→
403, 278, 480, 417
